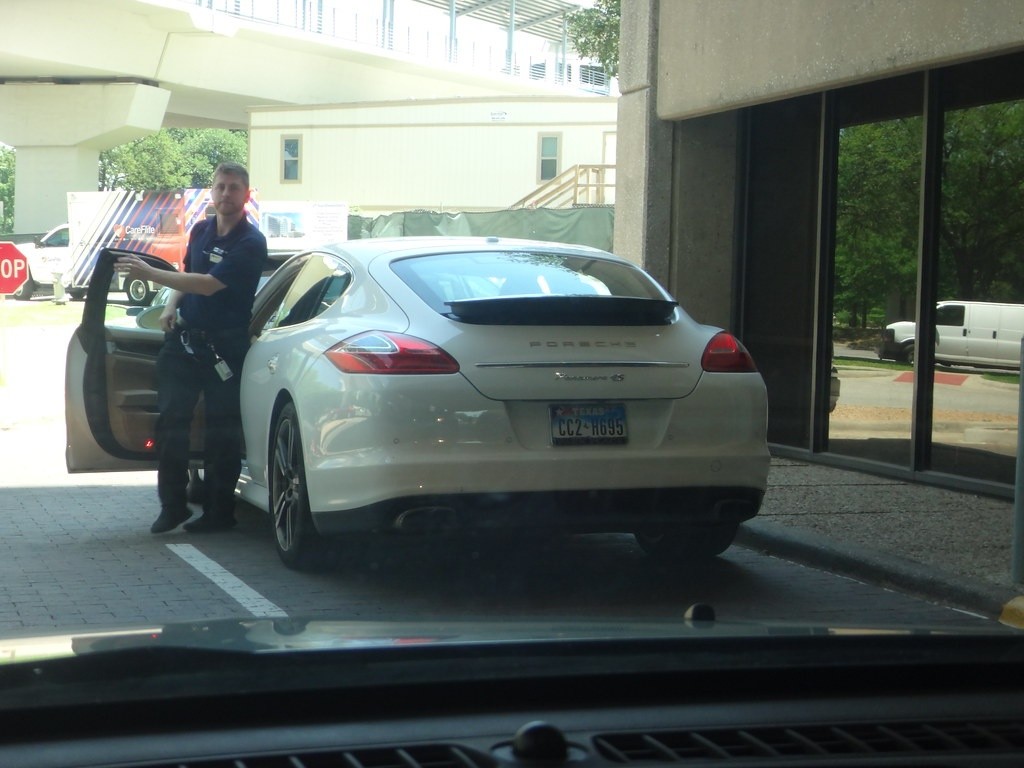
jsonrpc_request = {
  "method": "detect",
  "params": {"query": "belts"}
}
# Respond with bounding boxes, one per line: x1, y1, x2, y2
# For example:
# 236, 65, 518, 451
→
175, 311, 246, 342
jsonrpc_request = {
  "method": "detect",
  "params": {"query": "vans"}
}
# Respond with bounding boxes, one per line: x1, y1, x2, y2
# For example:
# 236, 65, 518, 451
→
876, 301, 1024, 367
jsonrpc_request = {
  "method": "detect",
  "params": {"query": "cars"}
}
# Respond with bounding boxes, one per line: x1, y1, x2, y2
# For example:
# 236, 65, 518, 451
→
126, 248, 300, 315
67, 236, 771, 573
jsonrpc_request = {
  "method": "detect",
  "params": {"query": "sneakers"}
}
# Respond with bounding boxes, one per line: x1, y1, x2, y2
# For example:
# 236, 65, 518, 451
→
183, 512, 240, 533
150, 502, 194, 534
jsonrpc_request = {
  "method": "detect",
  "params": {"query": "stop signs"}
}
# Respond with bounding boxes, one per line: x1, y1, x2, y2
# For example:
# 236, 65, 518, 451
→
0, 241, 28, 295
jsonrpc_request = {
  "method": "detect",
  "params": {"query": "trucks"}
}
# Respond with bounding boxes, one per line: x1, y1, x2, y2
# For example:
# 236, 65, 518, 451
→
15, 188, 262, 305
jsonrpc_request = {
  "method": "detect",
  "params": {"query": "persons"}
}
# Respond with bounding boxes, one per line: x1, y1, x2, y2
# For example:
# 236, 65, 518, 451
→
112, 161, 266, 533
